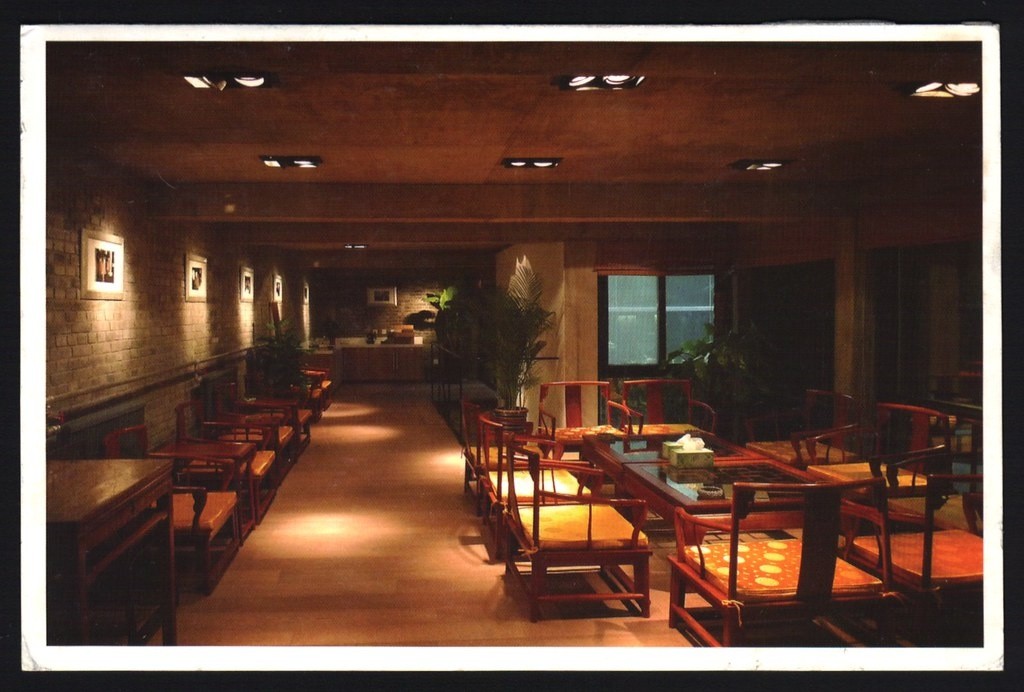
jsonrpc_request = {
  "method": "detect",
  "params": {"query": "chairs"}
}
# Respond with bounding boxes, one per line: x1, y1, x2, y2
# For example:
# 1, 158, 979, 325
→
101, 346, 331, 604
458, 364, 984, 649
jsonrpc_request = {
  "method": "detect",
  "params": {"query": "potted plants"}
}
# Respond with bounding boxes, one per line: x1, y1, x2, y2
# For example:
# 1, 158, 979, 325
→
459, 253, 562, 433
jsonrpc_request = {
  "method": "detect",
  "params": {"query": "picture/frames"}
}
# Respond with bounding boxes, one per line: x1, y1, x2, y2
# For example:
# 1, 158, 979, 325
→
81, 228, 125, 300
365, 286, 398, 307
240, 265, 255, 302
302, 286, 309, 304
185, 252, 207, 302
273, 274, 283, 302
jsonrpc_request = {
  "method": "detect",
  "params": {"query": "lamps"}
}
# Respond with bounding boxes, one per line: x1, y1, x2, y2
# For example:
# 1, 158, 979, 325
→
181, 68, 268, 94
560, 74, 646, 92
900, 80, 982, 100
733, 159, 790, 171
501, 158, 564, 169
259, 156, 321, 168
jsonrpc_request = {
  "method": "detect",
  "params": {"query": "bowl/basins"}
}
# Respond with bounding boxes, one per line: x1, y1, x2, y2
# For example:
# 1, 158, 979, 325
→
697, 486, 723, 496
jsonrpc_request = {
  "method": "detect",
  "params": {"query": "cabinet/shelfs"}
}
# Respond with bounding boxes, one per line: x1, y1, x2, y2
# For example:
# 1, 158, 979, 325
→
346, 346, 425, 379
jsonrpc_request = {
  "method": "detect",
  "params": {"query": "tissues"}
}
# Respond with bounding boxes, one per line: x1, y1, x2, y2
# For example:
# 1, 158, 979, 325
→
661, 432, 694, 461
670, 438, 716, 468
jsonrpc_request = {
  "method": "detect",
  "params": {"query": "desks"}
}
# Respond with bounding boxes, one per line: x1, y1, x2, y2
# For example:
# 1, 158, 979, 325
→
47, 457, 183, 645
